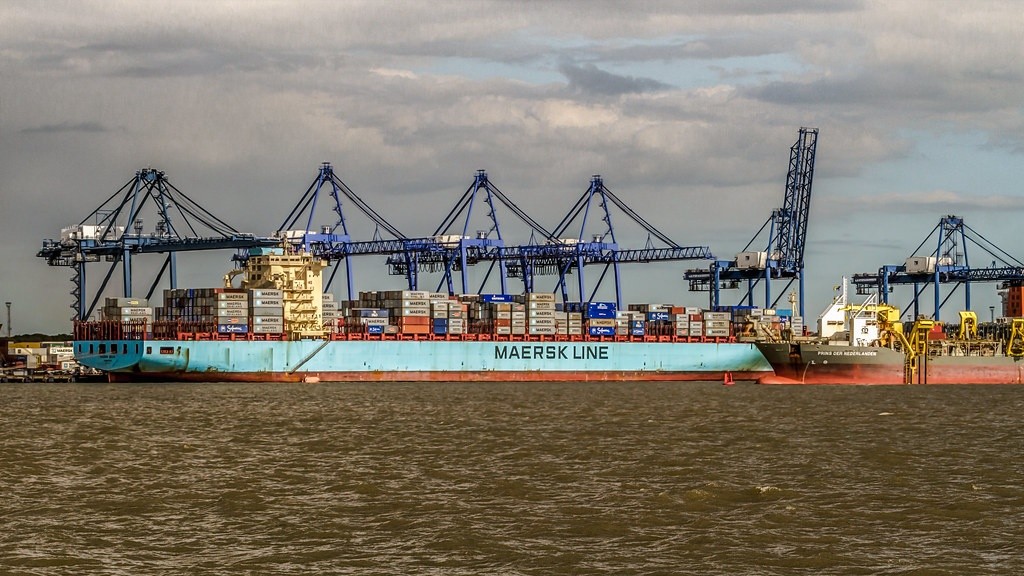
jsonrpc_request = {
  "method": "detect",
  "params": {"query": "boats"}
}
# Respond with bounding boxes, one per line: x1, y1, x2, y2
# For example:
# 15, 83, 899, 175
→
62, 318, 1024, 387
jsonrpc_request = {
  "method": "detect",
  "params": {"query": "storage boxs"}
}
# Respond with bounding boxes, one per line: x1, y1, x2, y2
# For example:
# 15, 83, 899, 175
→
10, 225, 1023, 373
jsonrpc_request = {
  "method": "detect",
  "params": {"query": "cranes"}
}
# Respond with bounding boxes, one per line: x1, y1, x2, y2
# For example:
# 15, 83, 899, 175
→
33, 164, 283, 323
680, 125, 818, 317
387, 166, 619, 297
248, 159, 442, 300
512, 172, 715, 308
848, 214, 1023, 319
